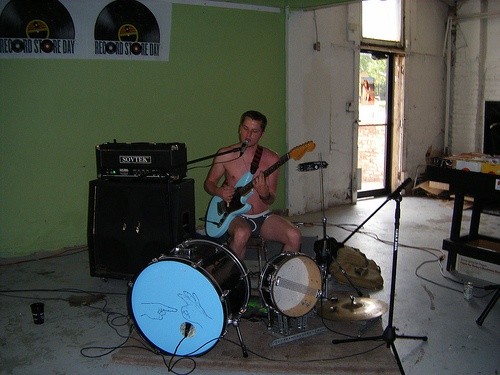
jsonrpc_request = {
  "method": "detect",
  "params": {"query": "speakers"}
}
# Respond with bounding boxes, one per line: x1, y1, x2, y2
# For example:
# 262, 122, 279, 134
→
88, 178, 195, 279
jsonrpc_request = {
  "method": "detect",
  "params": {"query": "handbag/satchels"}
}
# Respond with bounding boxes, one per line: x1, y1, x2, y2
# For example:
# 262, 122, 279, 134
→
330, 246, 384, 290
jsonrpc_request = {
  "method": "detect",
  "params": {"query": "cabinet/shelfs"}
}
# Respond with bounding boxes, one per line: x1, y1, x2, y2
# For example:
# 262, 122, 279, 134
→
424, 165, 500, 272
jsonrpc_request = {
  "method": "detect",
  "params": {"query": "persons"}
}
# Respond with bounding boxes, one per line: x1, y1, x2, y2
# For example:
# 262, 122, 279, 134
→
204, 110, 302, 259
361, 79, 374, 101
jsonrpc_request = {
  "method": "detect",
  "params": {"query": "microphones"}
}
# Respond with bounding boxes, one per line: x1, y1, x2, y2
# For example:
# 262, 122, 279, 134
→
240, 141, 249, 154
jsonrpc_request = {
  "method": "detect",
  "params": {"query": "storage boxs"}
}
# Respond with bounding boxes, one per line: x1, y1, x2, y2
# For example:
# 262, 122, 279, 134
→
456, 160, 500, 174
454, 251, 500, 285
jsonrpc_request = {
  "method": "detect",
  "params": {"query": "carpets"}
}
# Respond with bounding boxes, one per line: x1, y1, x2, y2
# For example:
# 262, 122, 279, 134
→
113, 235, 401, 375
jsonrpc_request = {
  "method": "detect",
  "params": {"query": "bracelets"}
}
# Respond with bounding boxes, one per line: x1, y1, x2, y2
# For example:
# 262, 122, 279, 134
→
259, 193, 272, 201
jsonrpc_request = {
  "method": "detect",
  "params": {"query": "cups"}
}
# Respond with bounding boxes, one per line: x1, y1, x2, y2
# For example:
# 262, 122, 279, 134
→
464, 281, 474, 300
31, 303, 45, 325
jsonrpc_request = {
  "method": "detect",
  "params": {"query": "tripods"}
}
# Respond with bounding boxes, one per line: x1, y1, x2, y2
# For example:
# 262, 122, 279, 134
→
332, 178, 427, 375
313, 152, 363, 298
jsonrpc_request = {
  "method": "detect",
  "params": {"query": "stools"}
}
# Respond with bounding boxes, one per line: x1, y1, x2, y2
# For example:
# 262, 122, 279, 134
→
228, 237, 270, 275
